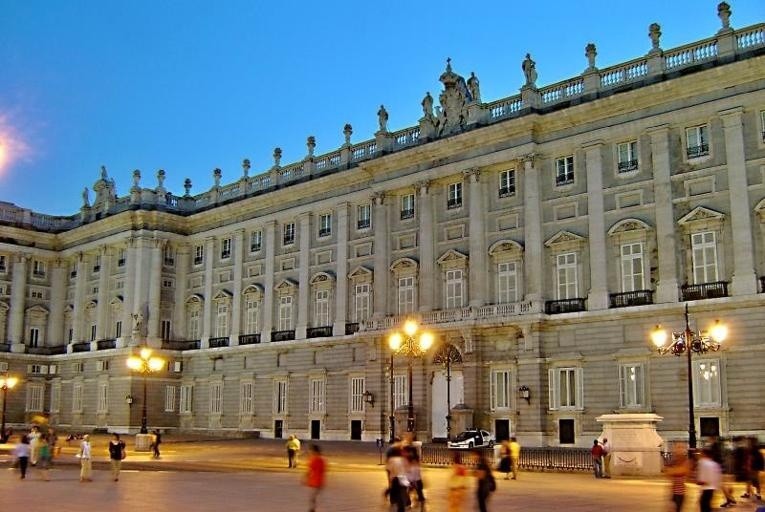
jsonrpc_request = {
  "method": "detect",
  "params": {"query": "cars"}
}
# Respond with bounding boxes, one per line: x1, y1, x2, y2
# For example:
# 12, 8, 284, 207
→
447, 426, 496, 453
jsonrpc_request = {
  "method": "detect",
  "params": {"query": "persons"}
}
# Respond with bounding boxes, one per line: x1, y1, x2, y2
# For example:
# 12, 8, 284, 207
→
522, 51, 536, 86
590, 439, 604, 478
661, 434, 765, 510
12, 422, 163, 483
469, 72, 480, 101
496, 440, 513, 480
377, 103, 387, 130
301, 442, 325, 512
285, 433, 299, 469
508, 436, 520, 481
377, 430, 497, 512
421, 91, 434, 118
294, 434, 302, 469
598, 437, 612, 479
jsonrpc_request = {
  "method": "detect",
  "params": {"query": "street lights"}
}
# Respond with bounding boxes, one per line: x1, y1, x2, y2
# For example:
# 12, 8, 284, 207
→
652, 301, 730, 448
0, 369, 21, 441
124, 346, 165, 453
387, 319, 436, 444
